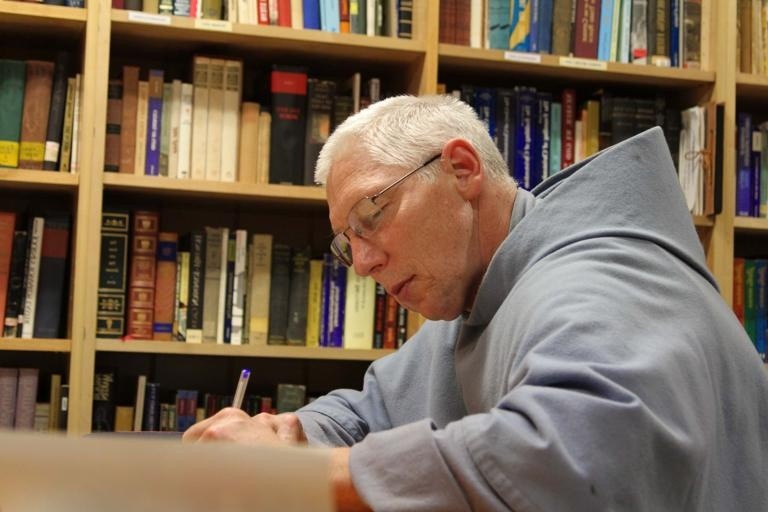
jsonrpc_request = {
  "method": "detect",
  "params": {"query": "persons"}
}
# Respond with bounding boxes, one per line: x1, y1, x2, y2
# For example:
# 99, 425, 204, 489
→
181, 92, 767, 511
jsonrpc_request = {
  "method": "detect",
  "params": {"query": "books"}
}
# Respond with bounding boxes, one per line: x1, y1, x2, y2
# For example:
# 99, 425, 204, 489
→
0, 1, 85, 435
441, 1, 724, 215
734, 2, 767, 360
91, 1, 415, 436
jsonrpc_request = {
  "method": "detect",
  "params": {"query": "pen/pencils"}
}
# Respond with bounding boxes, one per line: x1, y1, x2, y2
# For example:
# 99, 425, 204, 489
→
232, 369, 251, 409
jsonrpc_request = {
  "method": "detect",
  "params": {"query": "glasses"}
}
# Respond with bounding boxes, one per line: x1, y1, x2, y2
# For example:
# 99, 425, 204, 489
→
328, 154, 445, 270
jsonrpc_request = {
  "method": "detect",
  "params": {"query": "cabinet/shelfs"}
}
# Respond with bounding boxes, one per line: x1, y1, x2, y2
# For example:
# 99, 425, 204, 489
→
0, 0, 768, 443
